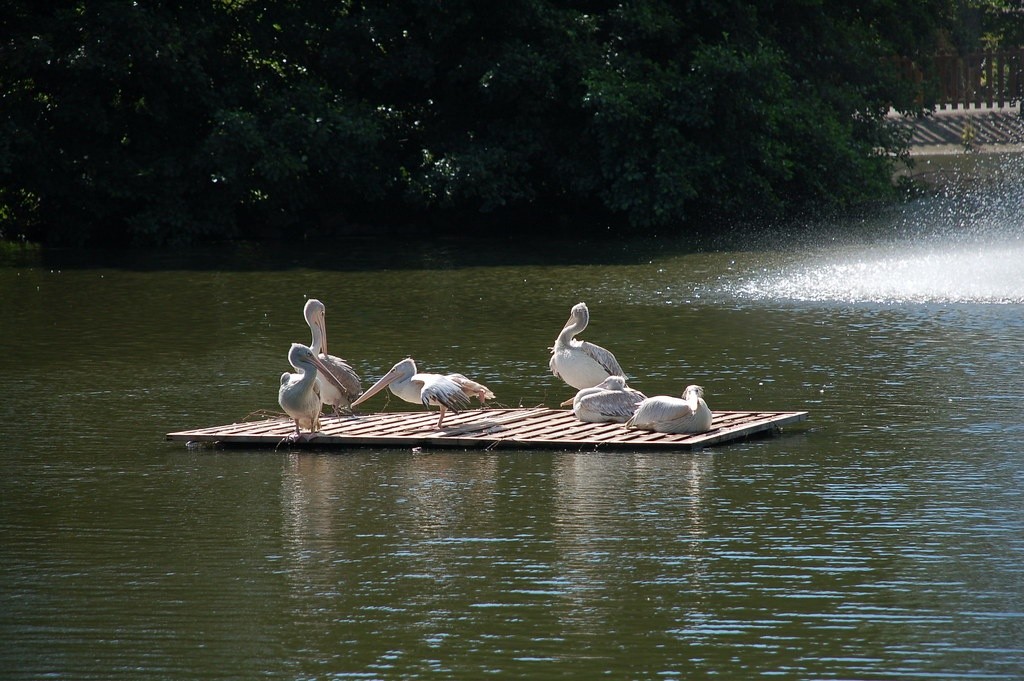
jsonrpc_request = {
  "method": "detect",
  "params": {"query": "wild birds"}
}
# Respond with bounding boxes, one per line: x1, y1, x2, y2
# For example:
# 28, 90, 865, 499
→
350, 358, 496, 428
278, 299, 363, 442
560, 376, 648, 424
627, 384, 711, 434
548, 302, 629, 387
